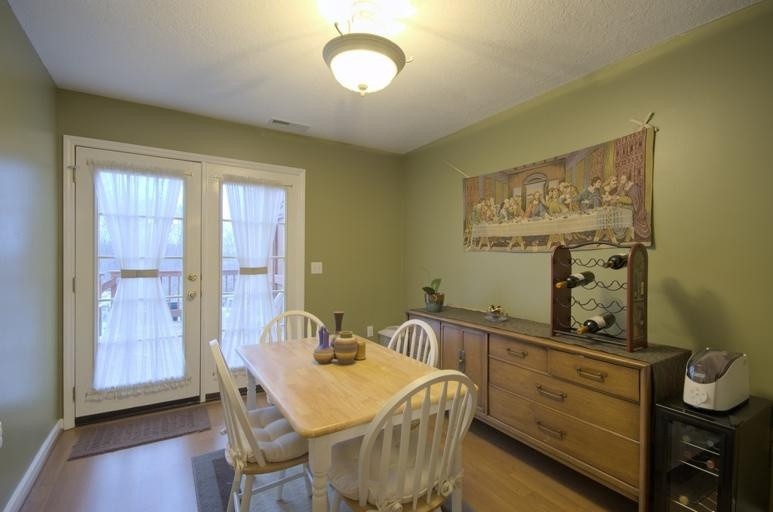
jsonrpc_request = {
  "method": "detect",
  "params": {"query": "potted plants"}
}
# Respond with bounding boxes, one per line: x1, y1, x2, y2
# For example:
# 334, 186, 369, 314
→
423, 278, 445, 313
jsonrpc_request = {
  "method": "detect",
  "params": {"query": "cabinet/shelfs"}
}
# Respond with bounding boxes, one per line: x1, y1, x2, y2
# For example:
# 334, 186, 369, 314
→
406, 306, 693, 511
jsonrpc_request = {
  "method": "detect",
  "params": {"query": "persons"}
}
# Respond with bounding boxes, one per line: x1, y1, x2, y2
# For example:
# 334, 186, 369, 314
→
472, 174, 648, 238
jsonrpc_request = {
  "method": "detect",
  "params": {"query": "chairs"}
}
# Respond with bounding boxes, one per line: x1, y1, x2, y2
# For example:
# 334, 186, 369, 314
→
209, 310, 479, 511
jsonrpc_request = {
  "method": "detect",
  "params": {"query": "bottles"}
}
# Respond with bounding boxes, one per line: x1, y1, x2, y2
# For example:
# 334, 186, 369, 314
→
602, 252, 629, 270
576, 311, 616, 335
555, 270, 595, 290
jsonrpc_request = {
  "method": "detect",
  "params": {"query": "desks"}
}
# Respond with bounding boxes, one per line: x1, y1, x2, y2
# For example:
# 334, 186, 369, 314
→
471, 206, 634, 250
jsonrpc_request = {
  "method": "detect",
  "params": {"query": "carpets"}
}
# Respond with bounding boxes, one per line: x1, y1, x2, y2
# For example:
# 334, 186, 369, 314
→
191, 446, 476, 511
69, 403, 212, 459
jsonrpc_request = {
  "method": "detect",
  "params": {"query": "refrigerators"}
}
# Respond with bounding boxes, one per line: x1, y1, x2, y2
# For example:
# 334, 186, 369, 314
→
653, 392, 773, 512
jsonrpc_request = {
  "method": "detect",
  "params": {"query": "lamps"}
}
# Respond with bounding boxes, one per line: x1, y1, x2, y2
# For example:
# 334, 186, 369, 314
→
323, 34, 406, 97
